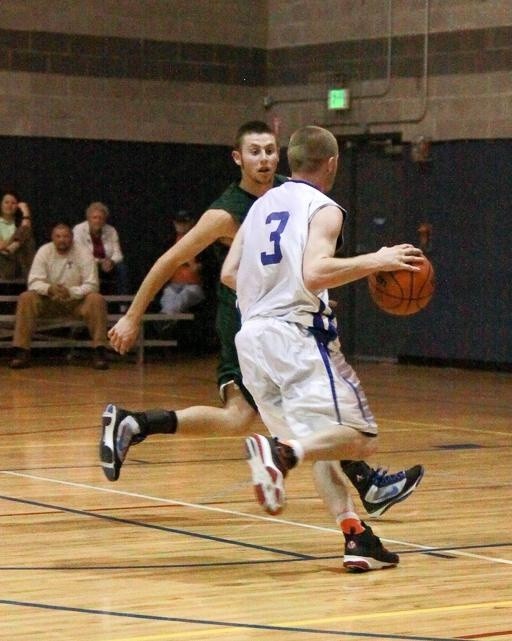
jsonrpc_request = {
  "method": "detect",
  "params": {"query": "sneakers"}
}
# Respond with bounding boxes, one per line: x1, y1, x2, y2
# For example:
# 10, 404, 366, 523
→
90, 352, 110, 370
241, 431, 299, 517
342, 519, 400, 572
98, 404, 150, 482
8, 349, 33, 370
355, 462, 425, 518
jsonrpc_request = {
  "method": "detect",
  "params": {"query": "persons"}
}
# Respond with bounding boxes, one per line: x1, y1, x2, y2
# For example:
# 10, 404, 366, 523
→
97, 120, 423, 522
147, 208, 219, 342
71, 199, 137, 313
0, 185, 32, 313
8, 221, 110, 369
214, 122, 423, 570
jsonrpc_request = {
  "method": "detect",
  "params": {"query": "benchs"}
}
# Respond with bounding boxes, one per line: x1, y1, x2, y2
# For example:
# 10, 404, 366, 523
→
2, 272, 194, 361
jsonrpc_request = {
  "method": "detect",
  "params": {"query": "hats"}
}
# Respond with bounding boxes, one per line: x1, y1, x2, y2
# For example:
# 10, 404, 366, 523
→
174, 209, 193, 224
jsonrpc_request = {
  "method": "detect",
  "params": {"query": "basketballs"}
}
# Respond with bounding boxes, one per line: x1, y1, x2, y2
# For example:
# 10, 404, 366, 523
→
369, 254, 435, 315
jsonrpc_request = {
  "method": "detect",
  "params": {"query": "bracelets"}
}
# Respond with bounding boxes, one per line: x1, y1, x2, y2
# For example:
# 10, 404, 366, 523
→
20, 216, 30, 220
